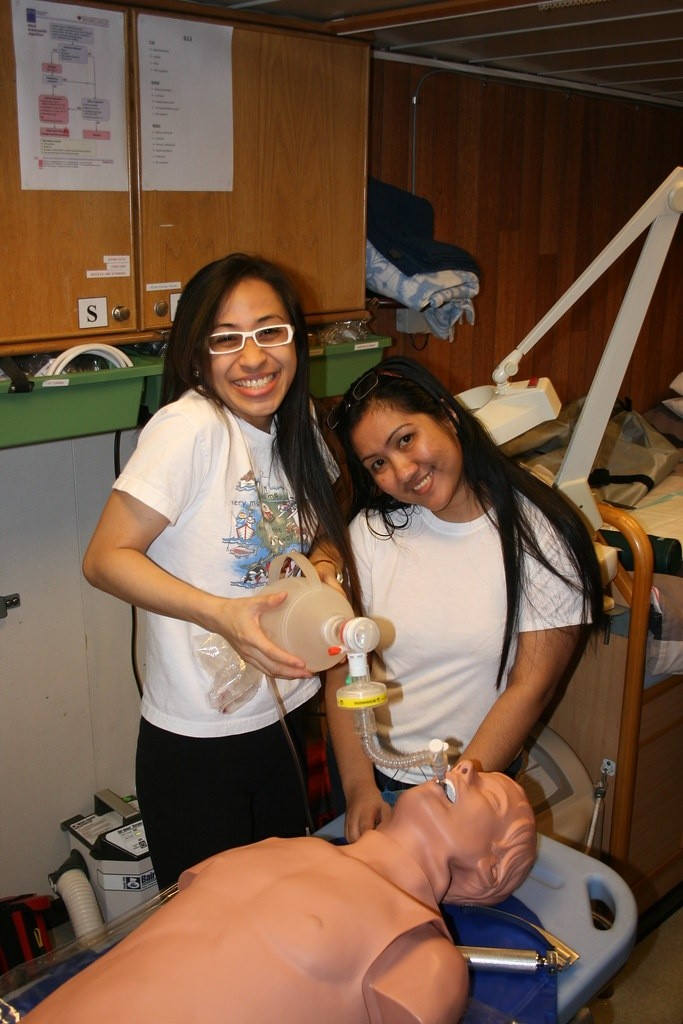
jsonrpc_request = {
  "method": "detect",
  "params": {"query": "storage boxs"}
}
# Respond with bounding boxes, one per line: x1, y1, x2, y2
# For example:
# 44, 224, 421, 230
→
141, 346, 184, 415
0, 353, 165, 449
307, 332, 393, 401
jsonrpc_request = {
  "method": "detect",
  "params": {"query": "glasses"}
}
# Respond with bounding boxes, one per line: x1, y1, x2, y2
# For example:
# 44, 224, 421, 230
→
206, 324, 295, 354
327, 371, 402, 429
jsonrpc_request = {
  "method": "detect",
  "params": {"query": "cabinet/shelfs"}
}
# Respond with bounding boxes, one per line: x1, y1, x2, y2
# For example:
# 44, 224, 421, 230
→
0, 0, 370, 356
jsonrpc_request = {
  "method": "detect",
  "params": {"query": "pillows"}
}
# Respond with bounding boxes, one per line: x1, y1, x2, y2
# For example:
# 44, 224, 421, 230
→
661, 372, 683, 421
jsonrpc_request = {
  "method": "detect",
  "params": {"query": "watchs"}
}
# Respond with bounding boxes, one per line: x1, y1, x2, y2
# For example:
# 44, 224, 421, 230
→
313, 560, 343, 584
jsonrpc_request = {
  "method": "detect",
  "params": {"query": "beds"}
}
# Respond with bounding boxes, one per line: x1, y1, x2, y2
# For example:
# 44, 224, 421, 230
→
517, 368, 683, 999
1, 788, 638, 1023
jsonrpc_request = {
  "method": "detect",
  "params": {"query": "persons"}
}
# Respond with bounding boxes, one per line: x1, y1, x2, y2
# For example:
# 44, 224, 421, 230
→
81, 251, 387, 902
325, 357, 606, 843
14, 759, 537, 1024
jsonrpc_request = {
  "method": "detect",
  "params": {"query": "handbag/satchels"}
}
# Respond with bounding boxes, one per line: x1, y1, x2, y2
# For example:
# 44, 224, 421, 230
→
0, 894, 55, 997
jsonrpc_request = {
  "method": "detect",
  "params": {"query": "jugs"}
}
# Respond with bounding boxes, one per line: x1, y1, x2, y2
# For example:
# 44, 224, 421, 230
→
256, 552, 380, 674
